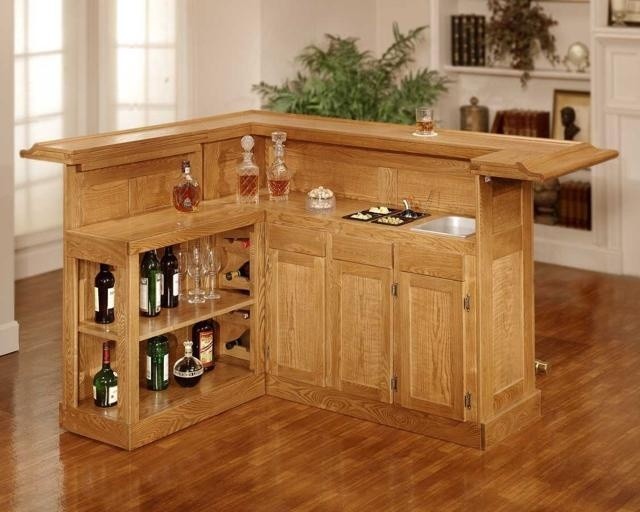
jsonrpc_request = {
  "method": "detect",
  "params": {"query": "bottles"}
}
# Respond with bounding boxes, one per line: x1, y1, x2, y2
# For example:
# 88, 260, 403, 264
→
226, 330, 250, 352
174, 342, 202, 388
304, 189, 336, 214
225, 260, 250, 282
145, 337, 170, 390
172, 160, 201, 214
237, 135, 261, 205
139, 244, 180, 317
192, 321, 218, 371
267, 131, 292, 200
92, 341, 118, 407
93, 263, 115, 324
460, 97, 488, 133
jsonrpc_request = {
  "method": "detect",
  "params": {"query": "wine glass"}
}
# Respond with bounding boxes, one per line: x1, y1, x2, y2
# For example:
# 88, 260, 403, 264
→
176, 247, 222, 303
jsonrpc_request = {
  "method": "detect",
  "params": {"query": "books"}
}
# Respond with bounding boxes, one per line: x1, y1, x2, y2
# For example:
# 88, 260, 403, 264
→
559, 178, 593, 231
449, 14, 503, 67
489, 108, 550, 139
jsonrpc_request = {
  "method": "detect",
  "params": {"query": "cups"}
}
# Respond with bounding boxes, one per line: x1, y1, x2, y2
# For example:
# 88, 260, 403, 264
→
415, 107, 434, 136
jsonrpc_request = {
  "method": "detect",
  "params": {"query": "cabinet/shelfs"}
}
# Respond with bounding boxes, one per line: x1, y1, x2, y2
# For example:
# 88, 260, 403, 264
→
264, 208, 542, 448
429, 0, 591, 273
62, 205, 266, 451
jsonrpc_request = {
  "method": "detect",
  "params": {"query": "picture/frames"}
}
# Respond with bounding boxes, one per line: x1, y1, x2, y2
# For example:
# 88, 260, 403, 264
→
550, 88, 590, 163
607, 0, 640, 28
